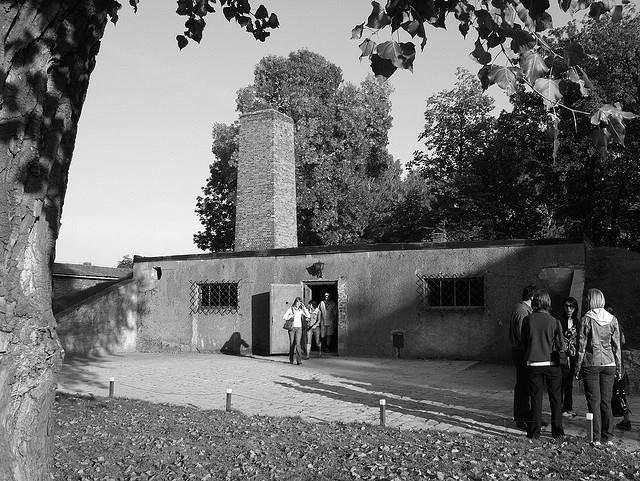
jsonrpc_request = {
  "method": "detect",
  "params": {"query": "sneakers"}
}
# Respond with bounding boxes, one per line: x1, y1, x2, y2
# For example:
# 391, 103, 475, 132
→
568, 409, 576, 415
562, 411, 572, 416
615, 419, 632, 430
325, 347, 330, 352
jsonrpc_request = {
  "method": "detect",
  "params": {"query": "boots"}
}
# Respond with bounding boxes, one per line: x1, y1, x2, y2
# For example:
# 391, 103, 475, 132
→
316, 341, 322, 357
305, 343, 311, 360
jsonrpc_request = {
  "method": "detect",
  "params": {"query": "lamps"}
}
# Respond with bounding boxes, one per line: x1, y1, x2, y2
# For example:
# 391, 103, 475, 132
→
314, 261, 324, 278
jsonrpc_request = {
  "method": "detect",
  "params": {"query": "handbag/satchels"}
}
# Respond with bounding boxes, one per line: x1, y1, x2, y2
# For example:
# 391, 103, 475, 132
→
558, 349, 568, 367
283, 307, 294, 331
612, 378, 628, 417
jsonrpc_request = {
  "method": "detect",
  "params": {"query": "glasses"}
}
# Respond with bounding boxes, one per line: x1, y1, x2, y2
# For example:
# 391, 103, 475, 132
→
565, 303, 575, 309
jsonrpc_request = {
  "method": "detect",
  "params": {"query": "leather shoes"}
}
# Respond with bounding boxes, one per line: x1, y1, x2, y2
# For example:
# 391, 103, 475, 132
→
516, 420, 528, 429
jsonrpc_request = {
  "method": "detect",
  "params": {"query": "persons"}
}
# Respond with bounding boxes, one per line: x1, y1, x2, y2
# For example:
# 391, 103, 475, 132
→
317, 292, 337, 353
304, 300, 322, 360
509, 285, 633, 447
283, 297, 311, 365
